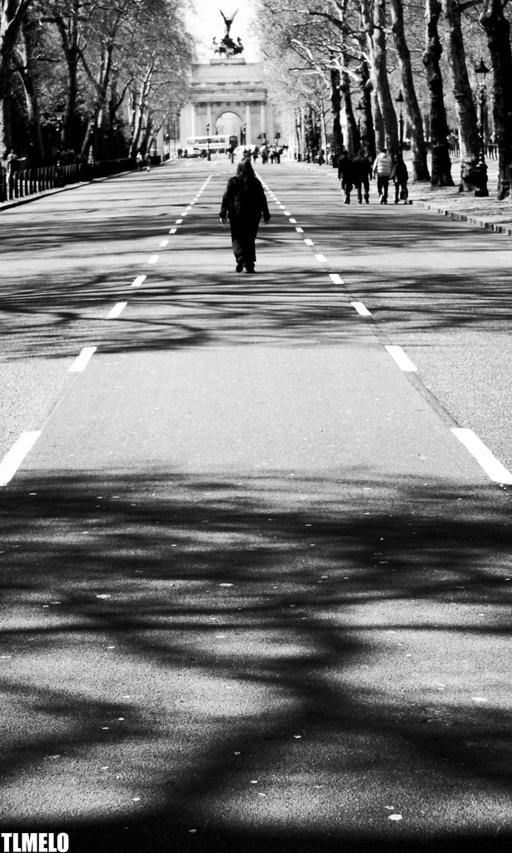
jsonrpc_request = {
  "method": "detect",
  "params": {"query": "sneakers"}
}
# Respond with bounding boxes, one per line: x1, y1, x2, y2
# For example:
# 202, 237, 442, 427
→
235, 256, 245, 273
246, 266, 254, 273
344, 195, 409, 204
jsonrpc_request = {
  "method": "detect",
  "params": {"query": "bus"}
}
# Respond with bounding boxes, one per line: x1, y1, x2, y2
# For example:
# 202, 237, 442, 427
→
186, 135, 238, 158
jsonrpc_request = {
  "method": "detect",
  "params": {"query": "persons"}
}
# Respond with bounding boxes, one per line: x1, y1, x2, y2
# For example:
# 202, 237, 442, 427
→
477, 132, 501, 160
223, 141, 285, 164
338, 149, 412, 203
136, 151, 145, 171
143, 151, 152, 169
219, 159, 273, 272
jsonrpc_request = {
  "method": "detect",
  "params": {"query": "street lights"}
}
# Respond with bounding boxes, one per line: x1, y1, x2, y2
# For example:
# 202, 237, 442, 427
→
205, 123, 211, 161
242, 121, 246, 146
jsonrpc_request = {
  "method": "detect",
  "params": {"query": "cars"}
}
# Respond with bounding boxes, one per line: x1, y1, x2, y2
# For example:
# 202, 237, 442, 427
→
181, 147, 202, 159
